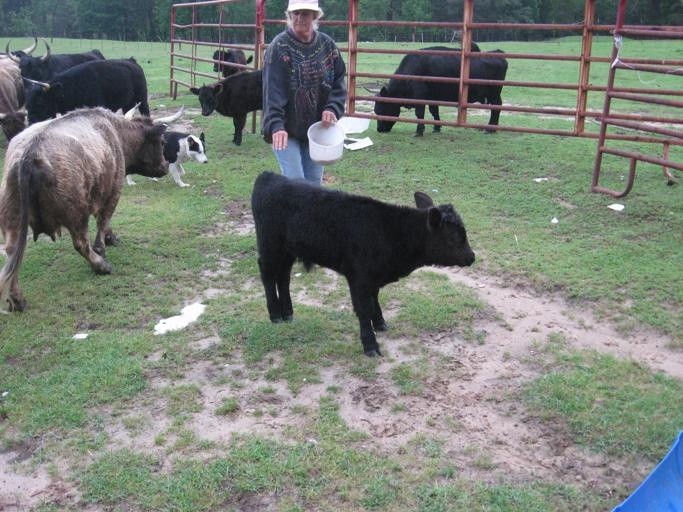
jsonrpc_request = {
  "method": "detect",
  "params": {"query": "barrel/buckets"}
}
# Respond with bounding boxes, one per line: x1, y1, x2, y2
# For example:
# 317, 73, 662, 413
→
306, 122, 347, 163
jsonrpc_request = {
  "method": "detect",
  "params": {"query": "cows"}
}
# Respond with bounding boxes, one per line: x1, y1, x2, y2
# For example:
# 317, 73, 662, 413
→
213, 48, 253, 78
251, 171, 475, 356
0, 101, 185, 314
189, 69, 263, 146
363, 41, 509, 138
0, 37, 151, 143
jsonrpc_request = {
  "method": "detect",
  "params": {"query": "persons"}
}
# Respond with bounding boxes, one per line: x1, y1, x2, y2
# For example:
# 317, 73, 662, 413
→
262, 1, 348, 183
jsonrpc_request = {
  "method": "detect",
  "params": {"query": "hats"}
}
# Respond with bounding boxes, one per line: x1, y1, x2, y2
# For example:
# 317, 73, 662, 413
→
287, 0, 324, 22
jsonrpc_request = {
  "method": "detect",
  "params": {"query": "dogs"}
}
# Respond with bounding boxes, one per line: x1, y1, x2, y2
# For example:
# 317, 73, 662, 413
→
125, 132, 207, 187
